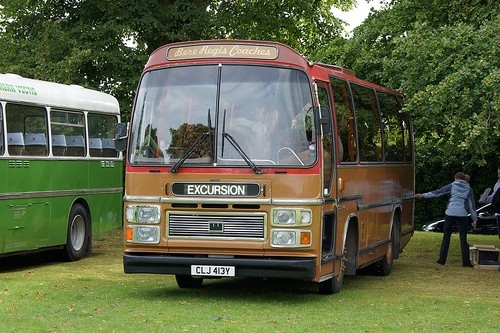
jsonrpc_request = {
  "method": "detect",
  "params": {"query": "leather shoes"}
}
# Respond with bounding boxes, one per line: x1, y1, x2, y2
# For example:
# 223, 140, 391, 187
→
437, 258, 445, 266
462, 262, 473, 268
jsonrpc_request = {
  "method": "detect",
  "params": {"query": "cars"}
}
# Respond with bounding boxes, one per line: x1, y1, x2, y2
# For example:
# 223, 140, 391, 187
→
421, 202, 498, 235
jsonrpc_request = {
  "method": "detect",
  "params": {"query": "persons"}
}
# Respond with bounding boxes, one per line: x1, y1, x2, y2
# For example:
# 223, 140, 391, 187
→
414, 171, 478, 267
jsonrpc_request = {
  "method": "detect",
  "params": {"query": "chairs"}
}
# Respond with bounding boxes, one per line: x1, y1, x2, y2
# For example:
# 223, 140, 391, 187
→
66, 135, 84, 156
89, 138, 104, 157
0, 132, 25, 155
23, 133, 47, 156
101, 139, 118, 157
51, 134, 67, 156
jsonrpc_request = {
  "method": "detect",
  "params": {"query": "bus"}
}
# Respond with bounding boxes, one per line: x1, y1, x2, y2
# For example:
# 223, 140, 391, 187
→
111, 38, 419, 295
0, 71, 125, 262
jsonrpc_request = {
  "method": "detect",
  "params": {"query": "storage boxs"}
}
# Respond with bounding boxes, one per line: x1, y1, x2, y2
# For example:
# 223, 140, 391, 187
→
469, 244, 500, 265
